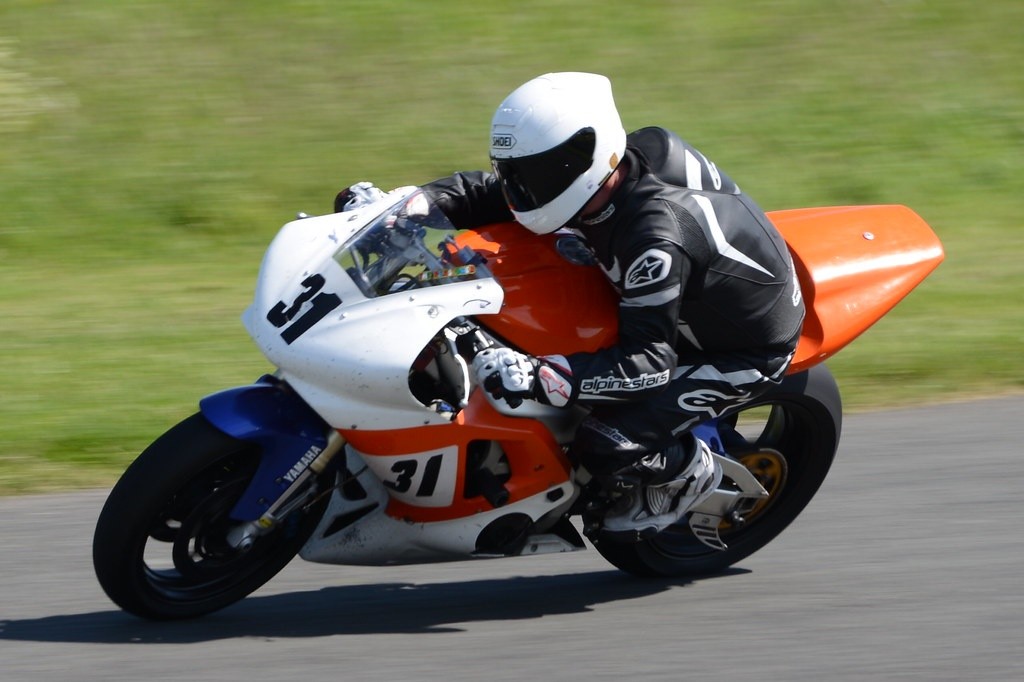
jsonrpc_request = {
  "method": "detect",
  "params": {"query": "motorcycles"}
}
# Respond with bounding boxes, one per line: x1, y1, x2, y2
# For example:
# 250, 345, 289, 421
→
94, 182, 946, 623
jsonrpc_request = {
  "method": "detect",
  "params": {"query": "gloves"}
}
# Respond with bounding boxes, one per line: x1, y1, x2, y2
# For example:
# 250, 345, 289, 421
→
471, 344, 576, 410
334, 182, 430, 226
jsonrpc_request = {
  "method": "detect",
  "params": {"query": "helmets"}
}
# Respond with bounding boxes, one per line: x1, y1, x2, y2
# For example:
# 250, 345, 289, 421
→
489, 72, 626, 236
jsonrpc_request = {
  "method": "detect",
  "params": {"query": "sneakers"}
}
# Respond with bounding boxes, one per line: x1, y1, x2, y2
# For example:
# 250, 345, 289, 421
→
600, 438, 722, 543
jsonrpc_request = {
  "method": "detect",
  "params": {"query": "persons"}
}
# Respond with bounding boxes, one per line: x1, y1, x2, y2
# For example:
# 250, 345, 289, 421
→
333, 70, 807, 540
